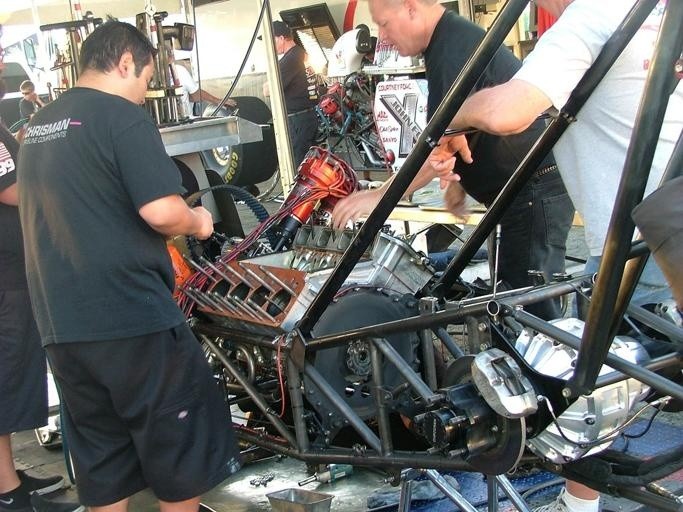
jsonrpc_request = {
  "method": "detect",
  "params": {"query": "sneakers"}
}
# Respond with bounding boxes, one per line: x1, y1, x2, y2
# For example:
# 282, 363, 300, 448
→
530, 486, 577, 512
0, 489, 86, 512
15, 469, 65, 496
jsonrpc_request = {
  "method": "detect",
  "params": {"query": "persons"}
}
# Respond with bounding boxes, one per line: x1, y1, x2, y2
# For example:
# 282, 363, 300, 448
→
14, 21, 243, 511
255, 20, 318, 178
428, 0, 681, 510
331, 0, 574, 320
0, 41, 87, 510
16, 76, 44, 120
167, 31, 237, 120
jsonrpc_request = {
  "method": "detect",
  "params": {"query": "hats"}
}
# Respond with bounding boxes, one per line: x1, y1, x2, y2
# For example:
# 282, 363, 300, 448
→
256, 20, 292, 40
19, 80, 36, 93
